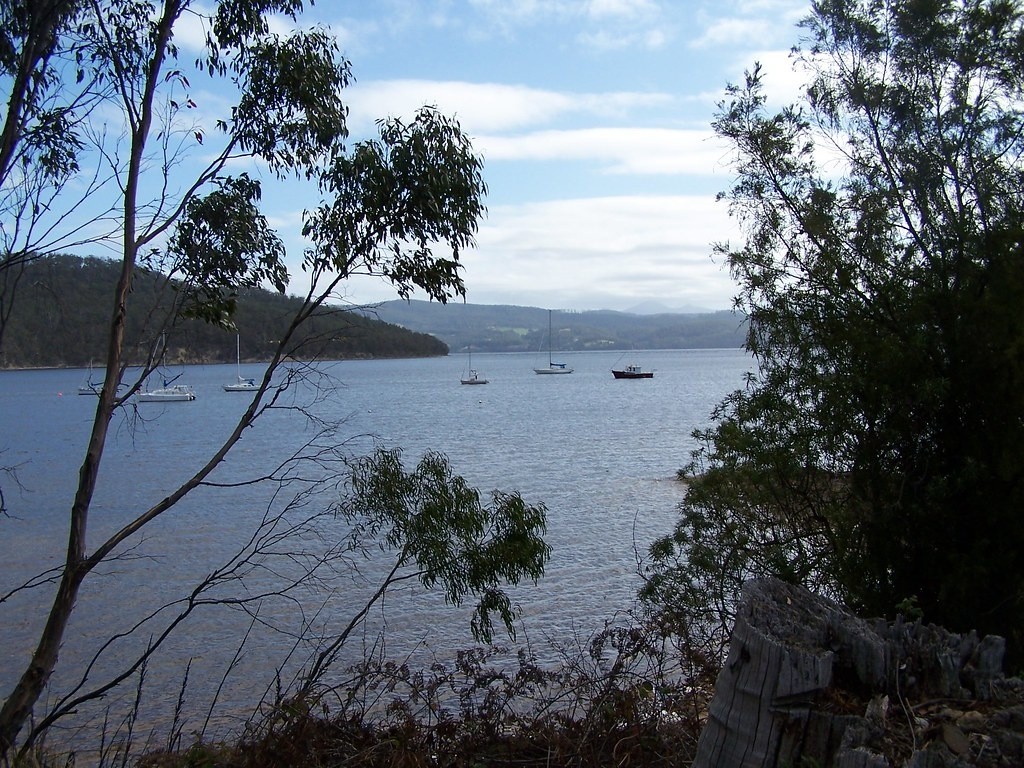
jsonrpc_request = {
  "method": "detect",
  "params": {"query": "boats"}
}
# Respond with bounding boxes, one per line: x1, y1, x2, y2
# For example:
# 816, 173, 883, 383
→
609, 353, 655, 378
457, 345, 490, 384
531, 310, 575, 374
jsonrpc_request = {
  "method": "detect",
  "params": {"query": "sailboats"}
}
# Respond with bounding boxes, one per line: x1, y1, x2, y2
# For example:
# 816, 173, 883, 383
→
220, 334, 261, 391
138, 331, 197, 402
77, 359, 103, 395
